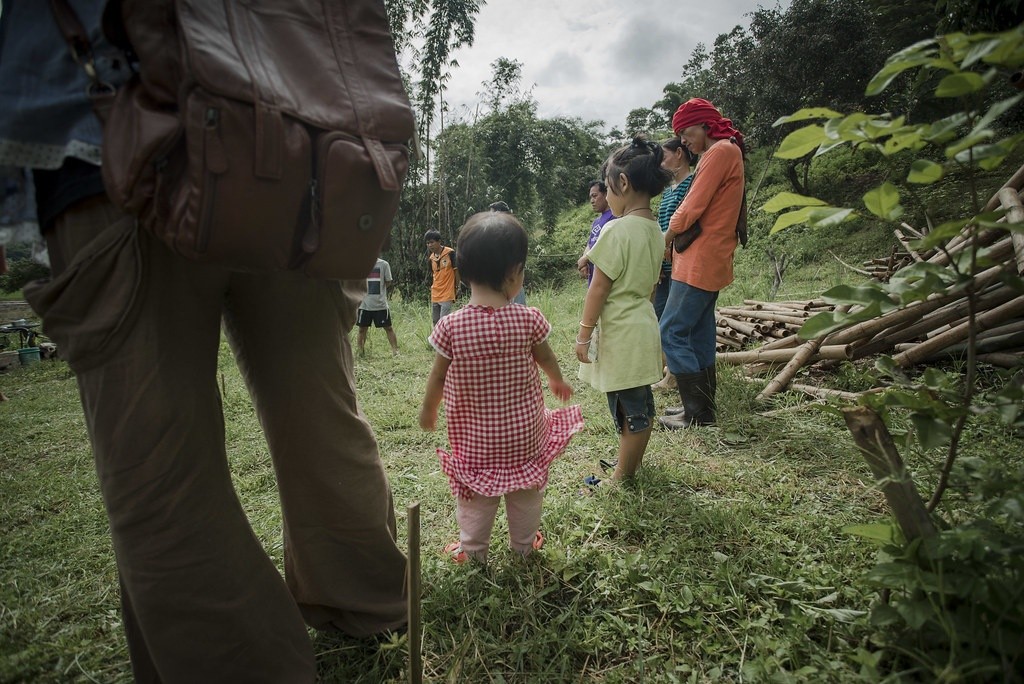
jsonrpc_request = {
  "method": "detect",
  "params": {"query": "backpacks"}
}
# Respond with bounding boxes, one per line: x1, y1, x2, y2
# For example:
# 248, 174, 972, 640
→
51, 1, 416, 282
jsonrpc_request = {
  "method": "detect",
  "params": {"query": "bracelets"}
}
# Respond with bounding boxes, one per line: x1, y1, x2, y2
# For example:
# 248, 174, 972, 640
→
579, 321, 598, 328
455, 285, 459, 288
576, 334, 591, 345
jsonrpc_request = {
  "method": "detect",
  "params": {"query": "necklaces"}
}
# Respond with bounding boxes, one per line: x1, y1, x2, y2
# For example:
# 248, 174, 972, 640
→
625, 207, 653, 217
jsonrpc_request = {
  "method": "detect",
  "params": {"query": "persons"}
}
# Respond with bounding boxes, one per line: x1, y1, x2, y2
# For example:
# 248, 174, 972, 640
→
649, 136, 697, 390
0, 0, 407, 684
419, 212, 584, 567
656, 99, 744, 432
489, 202, 526, 306
577, 180, 617, 290
424, 229, 461, 327
575, 137, 670, 494
355, 255, 399, 359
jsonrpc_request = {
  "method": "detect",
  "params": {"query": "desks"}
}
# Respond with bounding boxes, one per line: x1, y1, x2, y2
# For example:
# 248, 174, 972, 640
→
0, 323, 40, 349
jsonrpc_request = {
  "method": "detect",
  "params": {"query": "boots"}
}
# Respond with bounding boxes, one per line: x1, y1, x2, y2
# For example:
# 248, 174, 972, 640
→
657, 368, 716, 431
664, 363, 716, 414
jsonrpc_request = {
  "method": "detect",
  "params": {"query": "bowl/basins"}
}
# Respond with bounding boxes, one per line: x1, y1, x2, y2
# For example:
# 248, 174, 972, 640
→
11, 320, 25, 326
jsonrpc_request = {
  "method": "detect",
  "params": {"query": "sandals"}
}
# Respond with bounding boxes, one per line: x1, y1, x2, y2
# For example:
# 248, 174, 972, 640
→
444, 543, 466, 566
533, 531, 543, 550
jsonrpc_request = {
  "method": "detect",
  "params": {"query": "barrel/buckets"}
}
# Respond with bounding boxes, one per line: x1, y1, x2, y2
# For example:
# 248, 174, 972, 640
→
0, 351, 20, 367
17, 347, 41, 366
